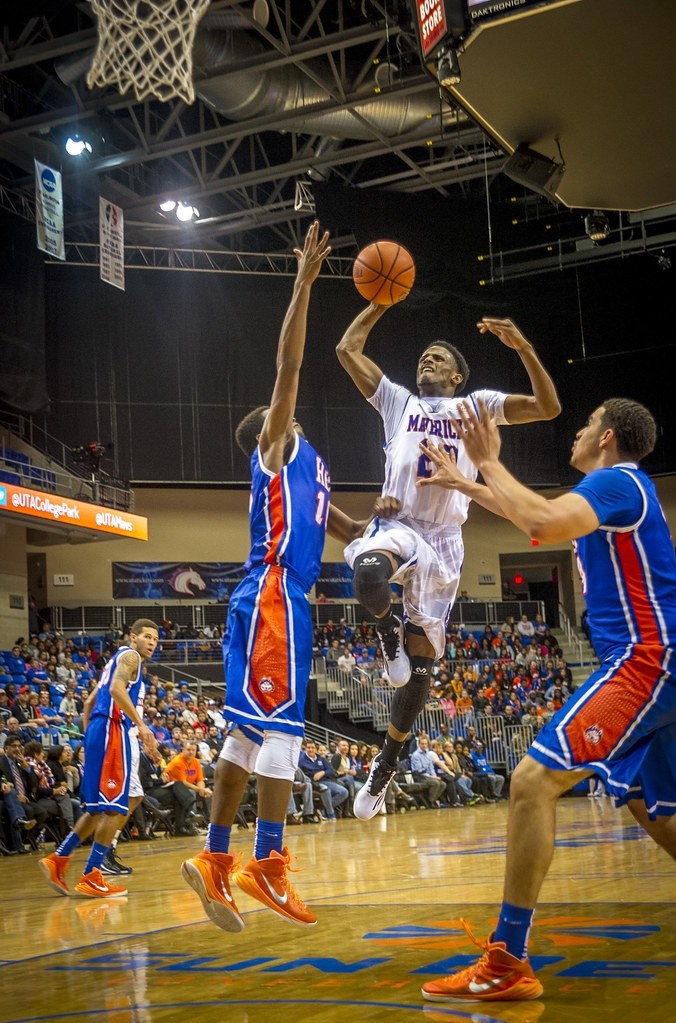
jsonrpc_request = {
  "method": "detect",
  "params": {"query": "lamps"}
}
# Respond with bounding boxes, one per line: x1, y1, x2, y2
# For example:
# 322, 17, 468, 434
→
433, 44, 461, 88
585, 211, 609, 240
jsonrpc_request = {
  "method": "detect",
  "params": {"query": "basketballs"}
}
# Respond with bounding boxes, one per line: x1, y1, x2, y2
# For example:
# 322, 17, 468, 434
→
353, 241, 415, 305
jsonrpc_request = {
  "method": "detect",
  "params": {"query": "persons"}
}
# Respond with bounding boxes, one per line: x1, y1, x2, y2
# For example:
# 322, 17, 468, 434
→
415, 398, 676, 1003
0, 618, 227, 855
457, 590, 468, 602
317, 593, 328, 603
586, 777, 596, 797
220, 594, 230, 603
334, 300, 561, 821
38, 617, 160, 898
179, 221, 400, 933
286, 614, 573, 825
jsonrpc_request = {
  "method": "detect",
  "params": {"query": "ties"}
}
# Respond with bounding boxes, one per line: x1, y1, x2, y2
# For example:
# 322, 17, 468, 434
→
12, 763, 24, 794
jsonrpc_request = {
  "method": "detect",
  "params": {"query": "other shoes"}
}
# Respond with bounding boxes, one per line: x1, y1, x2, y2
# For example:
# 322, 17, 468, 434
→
343, 792, 504, 819
602, 793, 606, 797
588, 793, 593, 796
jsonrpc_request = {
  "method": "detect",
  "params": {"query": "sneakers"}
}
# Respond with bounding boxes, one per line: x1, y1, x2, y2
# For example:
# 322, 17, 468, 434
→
422, 1001, 545, 1023
353, 752, 399, 821
45, 894, 69, 941
375, 614, 412, 687
233, 846, 317, 925
38, 852, 72, 896
181, 849, 246, 932
421, 918, 544, 1002
99, 848, 132, 875
74, 867, 128, 898
74, 897, 128, 929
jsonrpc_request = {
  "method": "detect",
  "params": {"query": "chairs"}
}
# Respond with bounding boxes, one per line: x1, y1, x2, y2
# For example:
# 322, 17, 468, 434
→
463, 630, 499, 642
0, 651, 35, 694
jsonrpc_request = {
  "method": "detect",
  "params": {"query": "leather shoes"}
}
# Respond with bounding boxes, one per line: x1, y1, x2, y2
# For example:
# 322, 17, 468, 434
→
0, 808, 336, 855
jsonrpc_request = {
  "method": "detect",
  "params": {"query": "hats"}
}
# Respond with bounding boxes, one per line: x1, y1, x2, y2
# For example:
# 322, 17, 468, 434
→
19, 687, 29, 694
167, 708, 175, 715
154, 711, 163, 719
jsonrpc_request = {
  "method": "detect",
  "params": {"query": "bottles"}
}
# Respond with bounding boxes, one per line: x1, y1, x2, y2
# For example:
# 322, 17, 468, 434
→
38, 830, 46, 851
